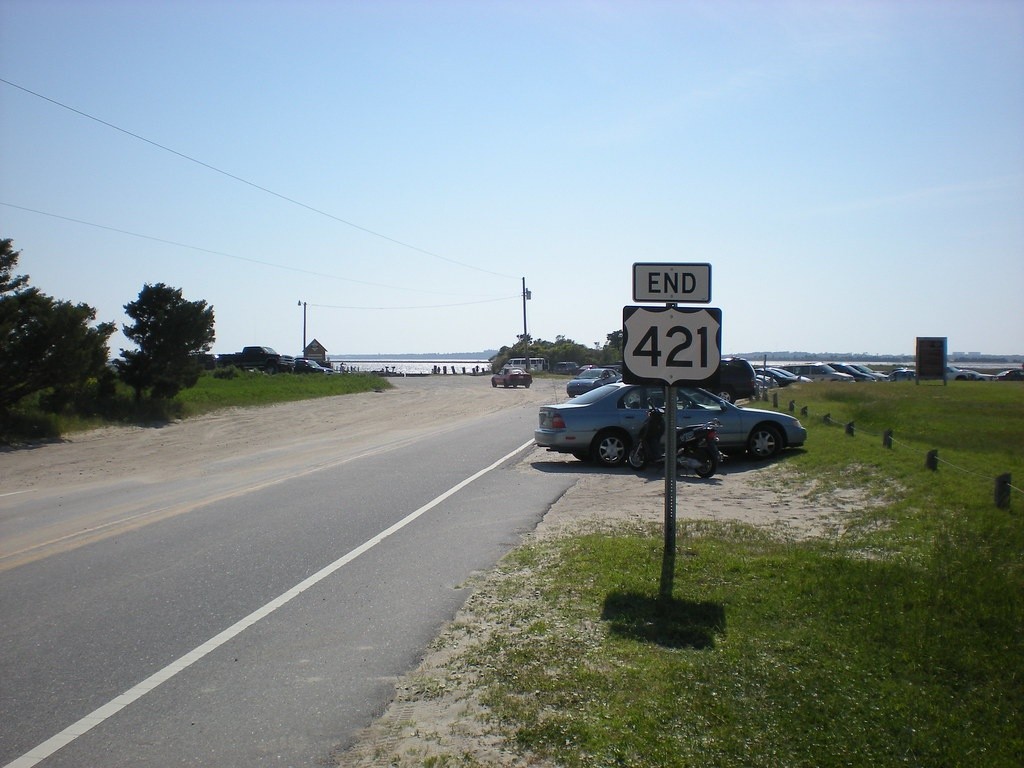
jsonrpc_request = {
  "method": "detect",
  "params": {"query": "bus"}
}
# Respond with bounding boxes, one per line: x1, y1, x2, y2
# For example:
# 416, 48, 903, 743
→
501, 357, 547, 372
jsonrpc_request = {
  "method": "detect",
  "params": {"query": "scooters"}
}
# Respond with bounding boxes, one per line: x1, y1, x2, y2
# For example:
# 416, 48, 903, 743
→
627, 394, 727, 479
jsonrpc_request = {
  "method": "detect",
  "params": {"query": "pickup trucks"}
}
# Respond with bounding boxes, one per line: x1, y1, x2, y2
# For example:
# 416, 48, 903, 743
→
218, 346, 297, 375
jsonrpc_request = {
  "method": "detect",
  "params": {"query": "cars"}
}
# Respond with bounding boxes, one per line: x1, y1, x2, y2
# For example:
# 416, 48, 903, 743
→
604, 365, 623, 373
532, 381, 809, 470
693, 356, 758, 407
490, 367, 533, 389
747, 362, 1024, 392
578, 363, 598, 375
565, 367, 624, 398
281, 355, 334, 376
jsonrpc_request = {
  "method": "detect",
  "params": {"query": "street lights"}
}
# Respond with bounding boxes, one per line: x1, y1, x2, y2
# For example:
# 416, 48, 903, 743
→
297, 300, 307, 359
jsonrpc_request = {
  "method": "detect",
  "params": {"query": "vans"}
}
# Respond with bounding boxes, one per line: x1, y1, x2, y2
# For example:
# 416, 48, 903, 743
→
555, 360, 578, 377
